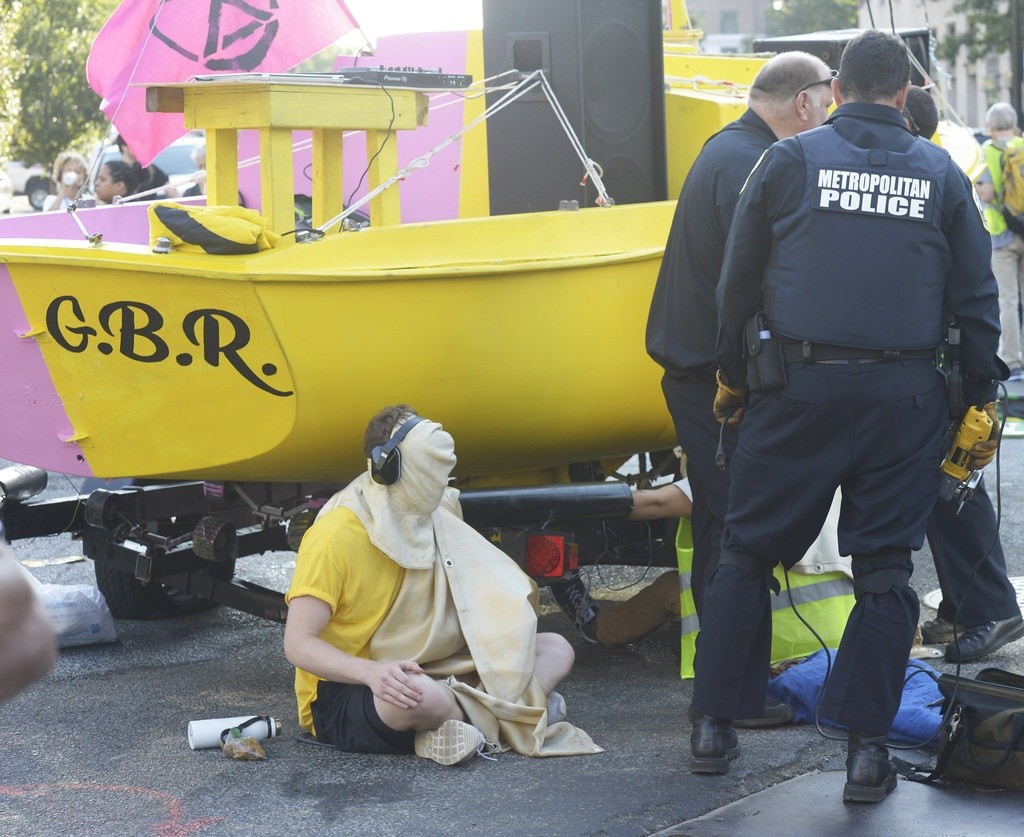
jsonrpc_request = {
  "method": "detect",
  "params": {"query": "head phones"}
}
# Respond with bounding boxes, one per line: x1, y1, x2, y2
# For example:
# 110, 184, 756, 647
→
367, 417, 426, 485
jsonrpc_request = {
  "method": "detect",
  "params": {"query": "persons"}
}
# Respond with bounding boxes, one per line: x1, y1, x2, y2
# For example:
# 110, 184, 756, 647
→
628, 477, 694, 520
691, 29, 1011, 803
0, 536, 58, 706
645, 50, 834, 728
930, 102, 1024, 381
283, 405, 575, 766
900, 85, 1024, 663
40, 134, 247, 211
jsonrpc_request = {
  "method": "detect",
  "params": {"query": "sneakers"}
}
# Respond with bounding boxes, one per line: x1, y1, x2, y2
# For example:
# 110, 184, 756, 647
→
414, 720, 498, 766
549, 575, 601, 643
547, 691, 566, 726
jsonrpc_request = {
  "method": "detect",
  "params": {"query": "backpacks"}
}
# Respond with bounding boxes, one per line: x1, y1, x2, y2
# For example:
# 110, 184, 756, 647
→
990, 142, 1024, 235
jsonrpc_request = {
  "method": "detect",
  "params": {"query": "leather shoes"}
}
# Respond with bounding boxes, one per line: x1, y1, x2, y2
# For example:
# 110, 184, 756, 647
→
945, 614, 1024, 663
689, 716, 740, 773
843, 748, 898, 802
920, 617, 965, 643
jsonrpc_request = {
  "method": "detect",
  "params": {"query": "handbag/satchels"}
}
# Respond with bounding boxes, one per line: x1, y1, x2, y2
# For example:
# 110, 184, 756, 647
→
891, 668, 1024, 791
13, 560, 118, 649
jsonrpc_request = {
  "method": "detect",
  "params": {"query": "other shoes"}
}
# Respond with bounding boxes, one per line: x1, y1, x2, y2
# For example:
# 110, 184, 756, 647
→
732, 695, 795, 727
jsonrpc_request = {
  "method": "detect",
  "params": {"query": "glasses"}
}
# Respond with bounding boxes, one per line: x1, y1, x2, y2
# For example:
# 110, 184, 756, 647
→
794, 70, 839, 99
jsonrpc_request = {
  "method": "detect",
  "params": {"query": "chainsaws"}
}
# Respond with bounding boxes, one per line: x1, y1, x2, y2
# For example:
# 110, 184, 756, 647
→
938, 404, 993, 517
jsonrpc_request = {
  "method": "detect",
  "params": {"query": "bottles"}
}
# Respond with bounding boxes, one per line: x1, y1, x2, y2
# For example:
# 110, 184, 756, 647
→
188, 716, 282, 750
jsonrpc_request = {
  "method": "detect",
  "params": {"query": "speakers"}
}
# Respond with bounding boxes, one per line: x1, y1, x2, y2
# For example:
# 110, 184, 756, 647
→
482, 0, 673, 216
